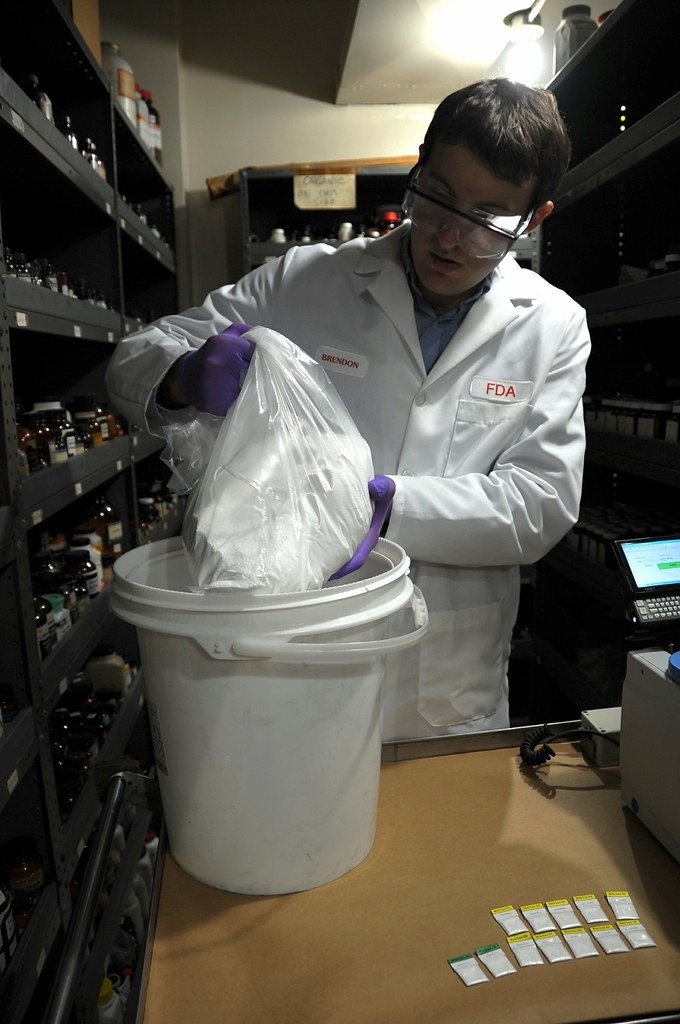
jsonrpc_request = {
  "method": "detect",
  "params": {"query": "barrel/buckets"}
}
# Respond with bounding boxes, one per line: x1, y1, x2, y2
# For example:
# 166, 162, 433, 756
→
108, 535, 429, 896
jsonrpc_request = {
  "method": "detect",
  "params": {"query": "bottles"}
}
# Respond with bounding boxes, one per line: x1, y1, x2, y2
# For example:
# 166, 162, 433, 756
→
13, 392, 125, 477
269, 212, 404, 241
582, 391, 680, 443
119, 192, 170, 249
565, 501, 680, 571
50, 645, 176, 819
140, 483, 180, 535
647, 253, 680, 277
24, 73, 105, 179
97, 829, 161, 1024
103, 40, 163, 166
31, 490, 126, 661
551, 4, 616, 77
8, 836, 52, 940
4, 246, 117, 312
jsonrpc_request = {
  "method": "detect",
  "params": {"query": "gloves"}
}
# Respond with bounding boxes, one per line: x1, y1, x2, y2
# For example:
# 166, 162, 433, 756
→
169, 322, 257, 415
331, 473, 396, 580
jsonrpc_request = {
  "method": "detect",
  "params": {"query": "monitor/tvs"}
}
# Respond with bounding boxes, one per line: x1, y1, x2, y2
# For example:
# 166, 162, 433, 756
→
611, 533, 680, 595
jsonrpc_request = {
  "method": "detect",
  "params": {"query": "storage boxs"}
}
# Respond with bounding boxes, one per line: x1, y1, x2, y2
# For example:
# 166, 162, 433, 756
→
62, 0, 102, 68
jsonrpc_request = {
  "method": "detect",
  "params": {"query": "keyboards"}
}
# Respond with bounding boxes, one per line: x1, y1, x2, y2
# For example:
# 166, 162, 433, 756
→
633, 595, 680, 623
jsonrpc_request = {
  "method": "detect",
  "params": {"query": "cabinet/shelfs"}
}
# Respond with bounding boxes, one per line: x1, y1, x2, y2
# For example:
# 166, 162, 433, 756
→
0, 0, 191, 1023
527, 1, 680, 727
238, 165, 532, 277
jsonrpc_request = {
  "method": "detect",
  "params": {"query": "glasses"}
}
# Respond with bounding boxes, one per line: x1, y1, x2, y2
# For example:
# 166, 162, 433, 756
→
399, 152, 537, 261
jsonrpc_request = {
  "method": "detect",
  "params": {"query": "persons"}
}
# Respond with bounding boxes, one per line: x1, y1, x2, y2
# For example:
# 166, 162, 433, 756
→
103, 80, 591, 743
